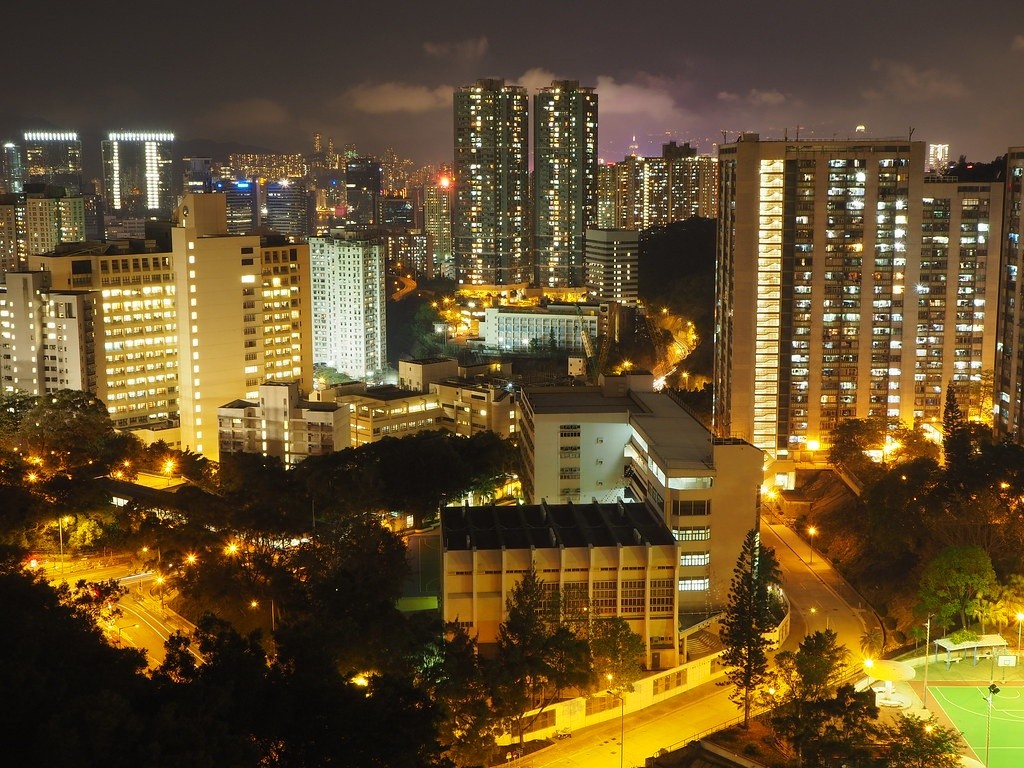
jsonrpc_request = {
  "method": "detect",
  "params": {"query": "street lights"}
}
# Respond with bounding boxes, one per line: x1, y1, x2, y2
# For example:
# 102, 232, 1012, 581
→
1017, 612, 1024, 662
157, 577, 164, 609
606, 690, 623, 768
809, 527, 815, 562
983, 684, 1000, 768
58, 516, 70, 574
770, 492, 774, 525
166, 467, 170, 485
168, 461, 173, 480
769, 688, 775, 718
864, 659, 874, 687
119, 623, 140, 650
920, 612, 935, 709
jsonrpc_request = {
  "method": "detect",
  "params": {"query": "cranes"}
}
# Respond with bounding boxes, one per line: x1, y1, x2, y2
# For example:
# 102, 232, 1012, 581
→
643, 303, 679, 392
574, 296, 619, 389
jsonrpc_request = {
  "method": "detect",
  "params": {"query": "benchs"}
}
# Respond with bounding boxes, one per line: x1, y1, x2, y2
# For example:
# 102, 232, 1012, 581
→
976, 654, 993, 660
873, 687, 895, 693
880, 699, 901, 707
944, 657, 963, 663
